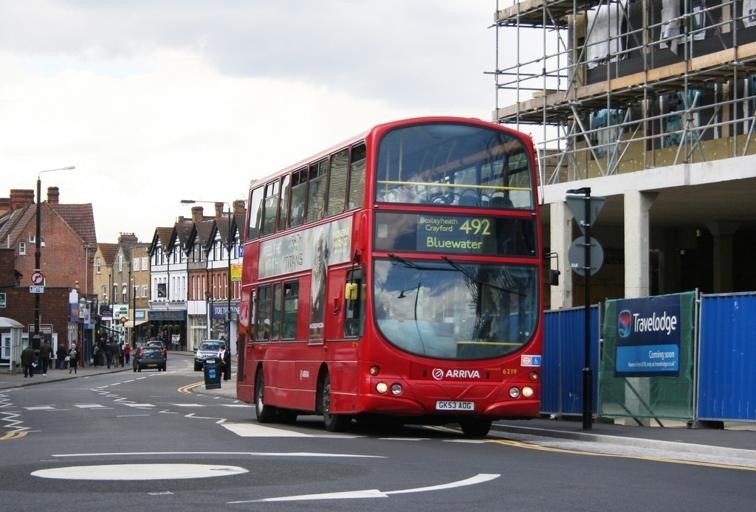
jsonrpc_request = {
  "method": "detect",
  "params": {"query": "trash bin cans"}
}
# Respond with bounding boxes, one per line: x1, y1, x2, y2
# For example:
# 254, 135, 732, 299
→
203, 357, 221, 390
31, 349, 41, 374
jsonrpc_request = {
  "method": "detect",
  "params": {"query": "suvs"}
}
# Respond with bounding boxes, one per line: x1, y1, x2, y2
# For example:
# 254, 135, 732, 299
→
130, 346, 162, 372
193, 339, 227, 372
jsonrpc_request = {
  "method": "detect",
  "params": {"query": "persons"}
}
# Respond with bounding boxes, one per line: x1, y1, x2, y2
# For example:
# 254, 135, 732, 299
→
159, 346, 167, 371
133, 345, 144, 372
218, 345, 231, 380
93, 337, 132, 368
21, 338, 80, 377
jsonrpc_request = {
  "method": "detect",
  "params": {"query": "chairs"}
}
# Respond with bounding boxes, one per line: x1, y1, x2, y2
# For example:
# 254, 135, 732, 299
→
428, 189, 513, 209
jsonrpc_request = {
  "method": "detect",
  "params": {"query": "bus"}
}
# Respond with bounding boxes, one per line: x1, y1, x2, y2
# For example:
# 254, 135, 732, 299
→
234, 115, 561, 439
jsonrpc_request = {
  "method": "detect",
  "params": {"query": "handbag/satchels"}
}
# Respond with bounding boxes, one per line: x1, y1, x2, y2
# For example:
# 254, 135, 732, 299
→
64, 356, 70, 361
90, 358, 94, 364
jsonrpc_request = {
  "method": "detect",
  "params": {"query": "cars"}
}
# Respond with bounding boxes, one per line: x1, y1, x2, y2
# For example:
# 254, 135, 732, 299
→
146, 341, 166, 349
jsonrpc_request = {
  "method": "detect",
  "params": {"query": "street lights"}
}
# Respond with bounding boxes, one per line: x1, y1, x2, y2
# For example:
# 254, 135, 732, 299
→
179, 198, 232, 381
32, 165, 77, 348
